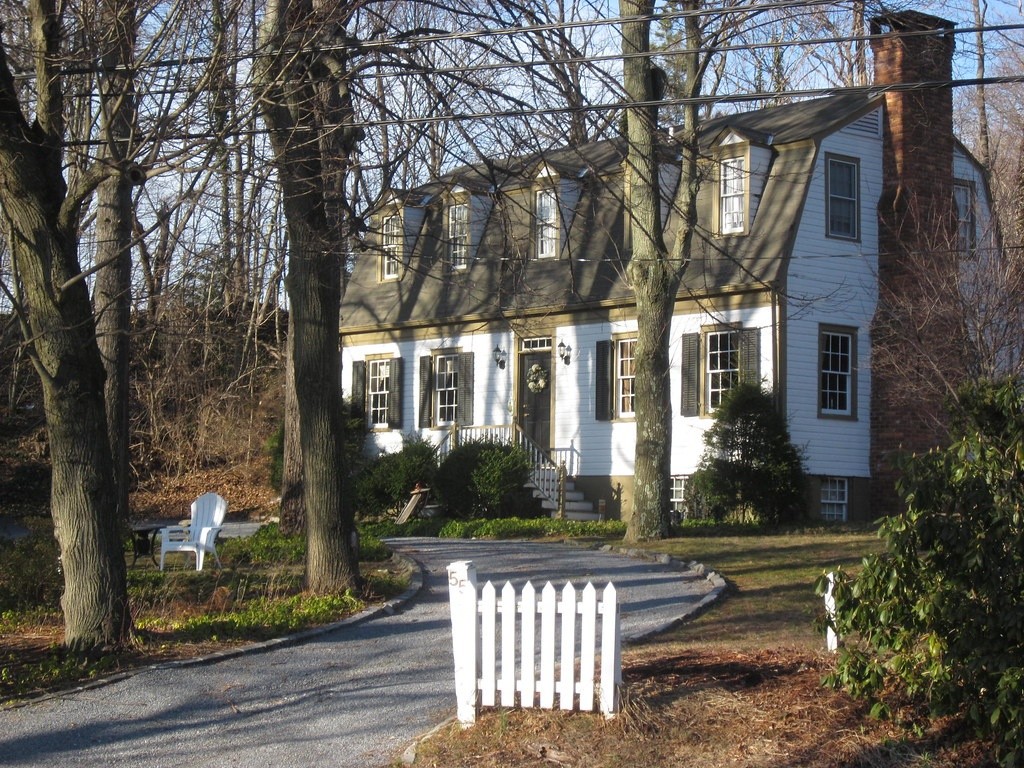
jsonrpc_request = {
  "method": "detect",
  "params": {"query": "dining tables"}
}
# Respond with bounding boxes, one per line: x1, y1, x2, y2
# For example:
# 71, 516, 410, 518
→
120, 523, 167, 570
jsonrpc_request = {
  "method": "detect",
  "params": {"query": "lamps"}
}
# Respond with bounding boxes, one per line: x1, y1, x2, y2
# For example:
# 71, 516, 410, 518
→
557, 338, 570, 365
492, 344, 505, 370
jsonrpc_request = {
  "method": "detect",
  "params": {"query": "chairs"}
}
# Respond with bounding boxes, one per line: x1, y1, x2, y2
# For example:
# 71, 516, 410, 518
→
159, 493, 228, 572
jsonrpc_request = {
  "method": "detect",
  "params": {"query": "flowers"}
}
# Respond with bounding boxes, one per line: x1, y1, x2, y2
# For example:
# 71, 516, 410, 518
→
526, 364, 548, 393
